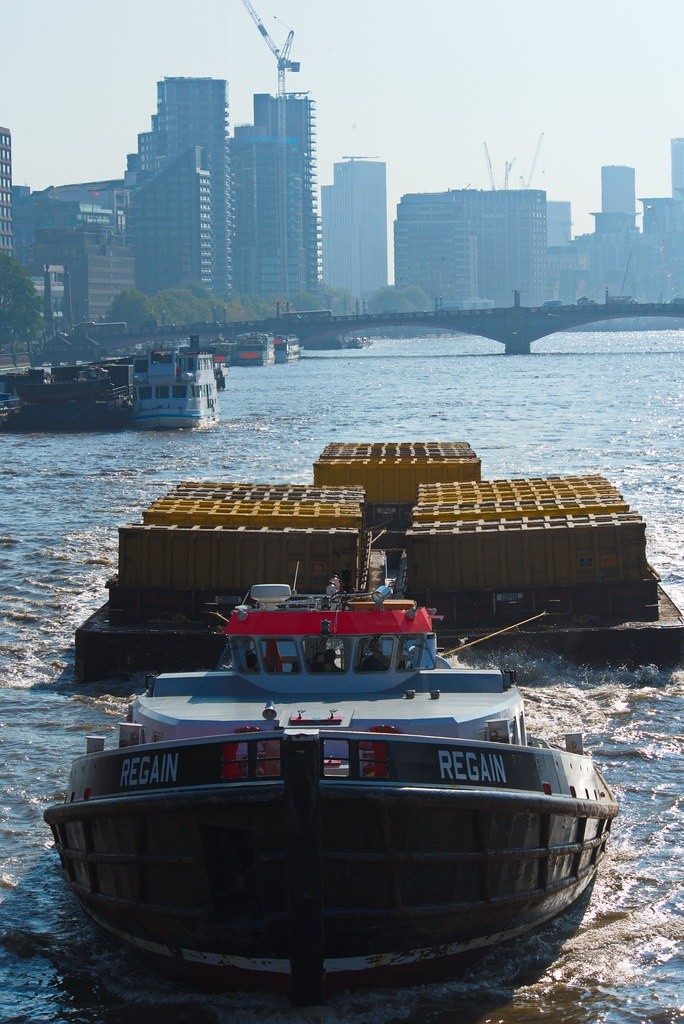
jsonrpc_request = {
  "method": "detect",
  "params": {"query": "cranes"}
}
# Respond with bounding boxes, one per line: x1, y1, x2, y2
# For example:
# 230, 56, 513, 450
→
243, 0, 302, 95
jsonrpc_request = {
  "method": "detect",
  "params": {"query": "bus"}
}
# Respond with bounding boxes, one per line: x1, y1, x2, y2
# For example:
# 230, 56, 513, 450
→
281, 311, 333, 321
70, 321, 128, 335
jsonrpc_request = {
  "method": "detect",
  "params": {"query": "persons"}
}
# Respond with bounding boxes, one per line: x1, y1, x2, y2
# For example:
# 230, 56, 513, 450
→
321, 649, 343, 673
360, 638, 392, 671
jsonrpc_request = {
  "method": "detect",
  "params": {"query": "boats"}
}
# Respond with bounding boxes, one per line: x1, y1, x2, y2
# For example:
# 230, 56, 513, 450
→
0, 333, 371, 433
43, 580, 622, 1010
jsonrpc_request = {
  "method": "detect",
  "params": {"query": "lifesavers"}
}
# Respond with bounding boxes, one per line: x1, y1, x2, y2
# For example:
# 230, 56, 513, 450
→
223, 726, 270, 778
359, 725, 401, 775
